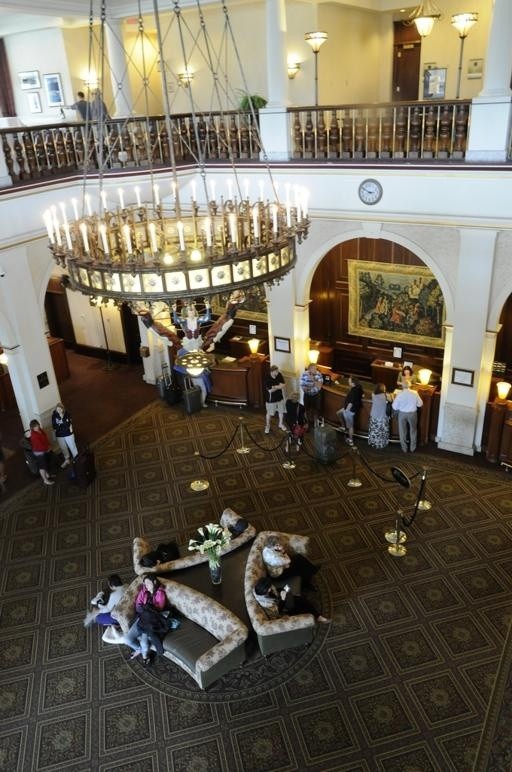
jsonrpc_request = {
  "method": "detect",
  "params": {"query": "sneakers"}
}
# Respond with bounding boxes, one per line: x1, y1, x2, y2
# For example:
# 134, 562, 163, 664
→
47, 475, 56, 478
44, 479, 54, 486
345, 438, 353, 445
62, 459, 70, 467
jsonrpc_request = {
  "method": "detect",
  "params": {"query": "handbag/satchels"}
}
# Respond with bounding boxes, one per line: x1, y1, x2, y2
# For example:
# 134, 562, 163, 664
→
41, 450, 57, 466
385, 400, 391, 416
292, 424, 304, 440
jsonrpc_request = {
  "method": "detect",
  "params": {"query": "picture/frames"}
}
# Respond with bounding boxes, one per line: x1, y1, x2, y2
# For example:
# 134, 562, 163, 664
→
18, 70, 65, 113
451, 367, 475, 388
347, 257, 446, 350
273, 336, 290, 354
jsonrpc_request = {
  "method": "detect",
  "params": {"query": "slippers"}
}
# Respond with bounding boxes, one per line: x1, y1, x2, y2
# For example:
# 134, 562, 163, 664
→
143, 657, 151, 666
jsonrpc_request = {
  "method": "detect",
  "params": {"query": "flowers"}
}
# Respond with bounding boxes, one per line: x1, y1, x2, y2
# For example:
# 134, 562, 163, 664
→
188, 523, 232, 571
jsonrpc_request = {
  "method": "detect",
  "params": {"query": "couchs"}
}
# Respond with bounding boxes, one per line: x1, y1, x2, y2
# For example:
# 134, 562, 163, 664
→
110, 574, 250, 692
131, 508, 257, 576
244, 531, 315, 661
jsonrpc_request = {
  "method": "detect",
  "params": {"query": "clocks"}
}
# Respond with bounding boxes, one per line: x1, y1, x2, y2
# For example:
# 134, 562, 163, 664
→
358, 179, 383, 205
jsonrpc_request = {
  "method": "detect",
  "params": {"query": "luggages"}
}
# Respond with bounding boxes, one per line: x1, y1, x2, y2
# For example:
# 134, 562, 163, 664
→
156, 363, 176, 399
312, 416, 336, 465
74, 450, 96, 483
182, 376, 201, 415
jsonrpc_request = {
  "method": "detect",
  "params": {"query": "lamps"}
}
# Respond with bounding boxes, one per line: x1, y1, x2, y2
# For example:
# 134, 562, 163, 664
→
400, 0, 449, 38
286, 51, 303, 79
177, 64, 196, 91
497, 382, 512, 400
303, 30, 329, 149
309, 350, 320, 366
248, 337, 261, 366
418, 368, 432, 387
450, 11, 478, 99
40, 0, 310, 309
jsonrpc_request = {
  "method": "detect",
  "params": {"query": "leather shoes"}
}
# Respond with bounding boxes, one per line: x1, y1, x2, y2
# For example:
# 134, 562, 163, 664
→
317, 619, 331, 624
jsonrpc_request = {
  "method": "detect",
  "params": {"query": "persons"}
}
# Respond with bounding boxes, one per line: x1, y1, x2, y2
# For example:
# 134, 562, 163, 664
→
172, 298, 211, 336
173, 346, 189, 398
30, 420, 57, 485
91, 573, 130, 628
125, 574, 166, 667
139, 541, 180, 567
262, 536, 322, 592
63, 91, 91, 121
196, 519, 248, 546
91, 88, 111, 122
263, 363, 424, 454
52, 403, 79, 469
191, 375, 209, 408
252, 577, 333, 624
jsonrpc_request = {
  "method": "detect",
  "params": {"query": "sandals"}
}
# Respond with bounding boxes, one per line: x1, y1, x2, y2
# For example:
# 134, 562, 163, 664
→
124, 652, 133, 661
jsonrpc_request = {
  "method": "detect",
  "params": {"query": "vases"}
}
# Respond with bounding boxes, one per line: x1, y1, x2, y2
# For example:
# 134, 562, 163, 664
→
207, 559, 221, 584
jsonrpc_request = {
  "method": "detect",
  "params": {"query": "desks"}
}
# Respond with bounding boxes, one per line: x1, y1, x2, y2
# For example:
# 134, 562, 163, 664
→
229, 334, 267, 359
1, 336, 71, 385
